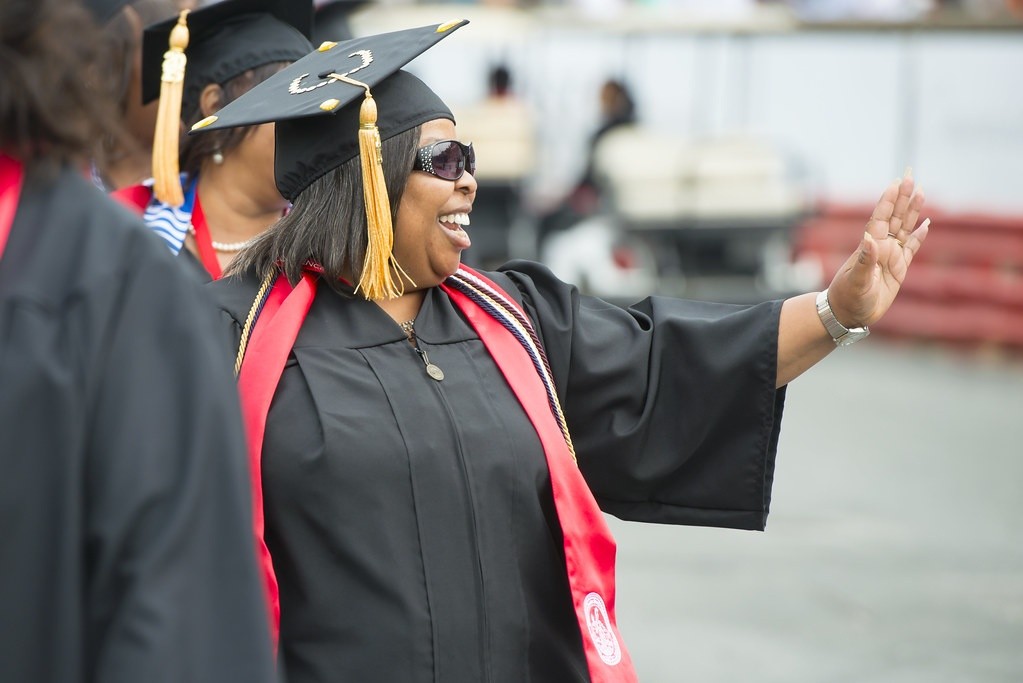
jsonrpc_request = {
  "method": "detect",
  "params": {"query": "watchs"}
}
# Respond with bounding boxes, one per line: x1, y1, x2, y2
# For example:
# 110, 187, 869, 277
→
816, 288, 871, 347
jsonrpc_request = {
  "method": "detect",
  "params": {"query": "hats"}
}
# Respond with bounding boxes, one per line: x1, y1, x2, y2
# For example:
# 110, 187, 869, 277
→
141, 0, 315, 208
312, 0, 364, 50
187, 19, 469, 297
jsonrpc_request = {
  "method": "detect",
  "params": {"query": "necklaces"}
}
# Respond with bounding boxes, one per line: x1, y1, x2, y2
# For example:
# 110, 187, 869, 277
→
189, 222, 253, 253
398, 317, 415, 334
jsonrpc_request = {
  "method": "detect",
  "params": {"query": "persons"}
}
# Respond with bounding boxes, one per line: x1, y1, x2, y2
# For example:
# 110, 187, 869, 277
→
193, 18, 931, 683
460, 66, 541, 268
105, 0, 375, 285
0, 0, 272, 683
537, 209, 659, 308
535, 79, 638, 249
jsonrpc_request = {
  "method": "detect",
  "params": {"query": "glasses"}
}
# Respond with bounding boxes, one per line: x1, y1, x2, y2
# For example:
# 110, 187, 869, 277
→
411, 138, 477, 181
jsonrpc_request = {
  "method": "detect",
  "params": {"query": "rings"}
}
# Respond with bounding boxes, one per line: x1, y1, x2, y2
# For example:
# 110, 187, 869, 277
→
895, 238, 903, 246
888, 232, 897, 237
